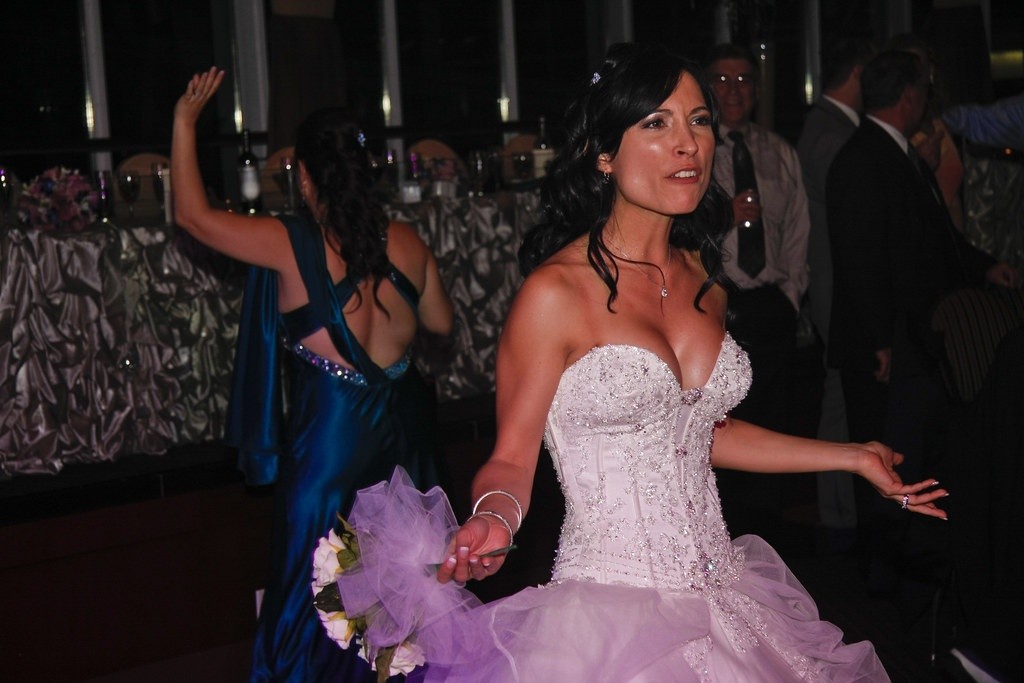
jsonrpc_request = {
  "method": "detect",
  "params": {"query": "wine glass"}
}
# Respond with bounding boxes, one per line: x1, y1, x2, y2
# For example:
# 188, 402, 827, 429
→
117, 176, 140, 219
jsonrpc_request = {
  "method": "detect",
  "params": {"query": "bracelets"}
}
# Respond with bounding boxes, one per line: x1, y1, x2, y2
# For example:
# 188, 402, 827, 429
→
466, 513, 513, 548
472, 490, 523, 531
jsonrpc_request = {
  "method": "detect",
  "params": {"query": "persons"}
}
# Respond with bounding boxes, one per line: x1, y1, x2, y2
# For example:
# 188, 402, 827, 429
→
683, 34, 1024, 683
165, 66, 453, 683
406, 40, 949, 683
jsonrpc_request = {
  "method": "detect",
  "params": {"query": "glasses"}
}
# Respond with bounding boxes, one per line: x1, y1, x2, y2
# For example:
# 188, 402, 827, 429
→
707, 73, 755, 83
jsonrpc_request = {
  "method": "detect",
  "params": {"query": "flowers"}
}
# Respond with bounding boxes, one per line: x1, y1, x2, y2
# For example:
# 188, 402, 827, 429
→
311, 512, 518, 683
15, 167, 101, 232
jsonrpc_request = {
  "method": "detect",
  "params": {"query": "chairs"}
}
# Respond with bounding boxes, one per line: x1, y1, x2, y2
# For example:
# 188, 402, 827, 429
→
259, 146, 295, 205
921, 279, 1023, 404
504, 135, 538, 182
862, 326, 1023, 634
381, 139, 470, 182
113, 154, 171, 203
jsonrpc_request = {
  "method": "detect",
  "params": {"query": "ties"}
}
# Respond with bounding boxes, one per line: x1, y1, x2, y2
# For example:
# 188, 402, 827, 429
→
728, 130, 765, 278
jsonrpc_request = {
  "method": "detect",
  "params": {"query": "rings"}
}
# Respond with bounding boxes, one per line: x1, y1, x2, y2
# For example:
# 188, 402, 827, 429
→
901, 493, 909, 509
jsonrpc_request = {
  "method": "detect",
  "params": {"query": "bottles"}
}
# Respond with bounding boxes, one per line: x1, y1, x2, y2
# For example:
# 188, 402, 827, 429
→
532, 117, 556, 177
233, 130, 265, 211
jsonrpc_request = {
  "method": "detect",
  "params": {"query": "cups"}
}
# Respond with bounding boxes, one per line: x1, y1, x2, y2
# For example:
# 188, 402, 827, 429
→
468, 151, 489, 191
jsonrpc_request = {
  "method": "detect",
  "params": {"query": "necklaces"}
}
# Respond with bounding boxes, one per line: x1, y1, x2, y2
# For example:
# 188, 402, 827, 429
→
601, 228, 670, 297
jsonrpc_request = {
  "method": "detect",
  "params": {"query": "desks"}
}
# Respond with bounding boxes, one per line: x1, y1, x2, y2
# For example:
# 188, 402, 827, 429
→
0, 193, 554, 500
959, 144, 1024, 267
